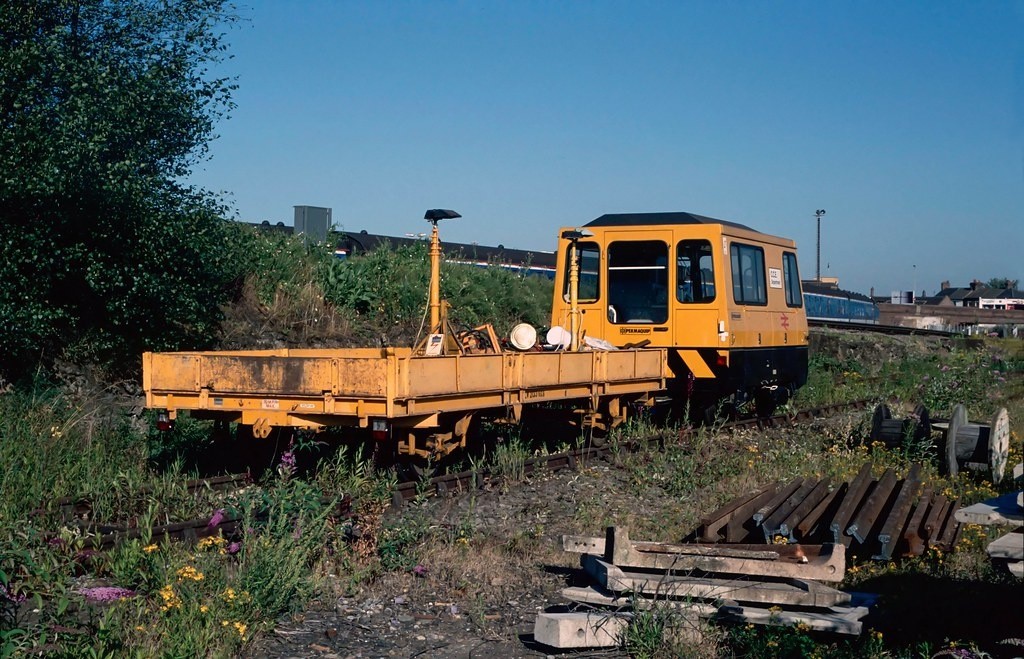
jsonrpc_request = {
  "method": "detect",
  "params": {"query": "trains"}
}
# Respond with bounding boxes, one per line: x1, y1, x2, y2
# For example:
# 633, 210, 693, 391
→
143, 212, 810, 477
231, 221, 878, 326
